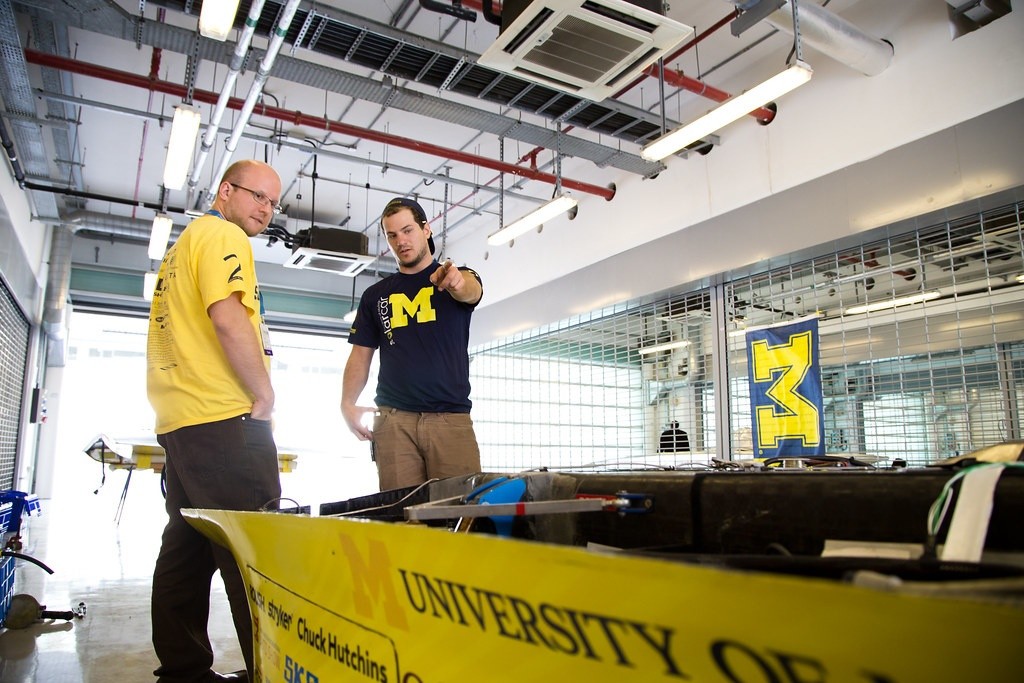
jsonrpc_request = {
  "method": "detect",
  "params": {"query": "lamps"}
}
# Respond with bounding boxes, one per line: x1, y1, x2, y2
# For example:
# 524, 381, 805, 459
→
162, 36, 202, 191
639, 313, 693, 354
196, 0, 241, 42
147, 190, 174, 262
640, 0, 814, 163
840, 266, 943, 315
143, 259, 158, 301
728, 282, 825, 339
487, 122, 578, 248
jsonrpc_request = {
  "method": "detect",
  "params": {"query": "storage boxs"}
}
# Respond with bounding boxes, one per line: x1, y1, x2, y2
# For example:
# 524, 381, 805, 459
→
0, 489, 43, 627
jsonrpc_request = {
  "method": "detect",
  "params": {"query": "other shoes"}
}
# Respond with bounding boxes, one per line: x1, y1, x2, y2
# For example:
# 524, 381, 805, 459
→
155, 669, 247, 683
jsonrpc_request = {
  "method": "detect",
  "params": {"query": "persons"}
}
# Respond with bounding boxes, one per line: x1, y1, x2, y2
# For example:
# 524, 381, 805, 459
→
339, 197, 484, 531
144, 159, 283, 682
658, 420, 690, 452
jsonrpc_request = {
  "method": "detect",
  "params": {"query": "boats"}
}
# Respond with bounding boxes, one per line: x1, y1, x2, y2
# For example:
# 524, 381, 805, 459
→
177, 443, 1024, 683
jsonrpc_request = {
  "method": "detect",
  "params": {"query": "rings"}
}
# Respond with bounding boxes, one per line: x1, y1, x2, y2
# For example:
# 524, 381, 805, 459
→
363, 435, 367, 437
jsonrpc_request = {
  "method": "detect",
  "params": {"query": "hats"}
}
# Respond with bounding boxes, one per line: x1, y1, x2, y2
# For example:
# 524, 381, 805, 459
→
381, 197, 436, 255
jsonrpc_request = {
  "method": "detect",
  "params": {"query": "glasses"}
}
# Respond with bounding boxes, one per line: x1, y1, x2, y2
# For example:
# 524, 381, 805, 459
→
230, 183, 282, 214
